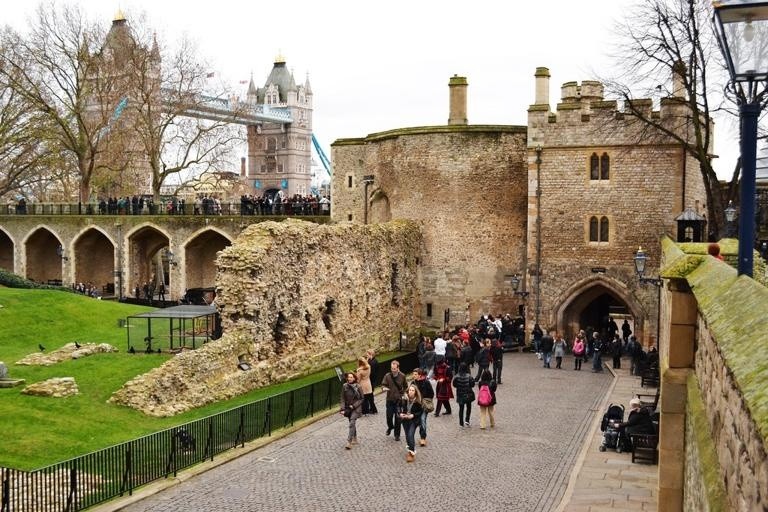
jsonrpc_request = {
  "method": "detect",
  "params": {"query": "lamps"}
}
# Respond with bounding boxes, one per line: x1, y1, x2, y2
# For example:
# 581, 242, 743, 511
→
634, 246, 663, 288
511, 273, 529, 299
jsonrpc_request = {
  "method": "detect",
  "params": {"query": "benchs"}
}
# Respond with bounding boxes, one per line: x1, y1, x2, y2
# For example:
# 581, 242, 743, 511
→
47, 279, 62, 286
102, 282, 114, 292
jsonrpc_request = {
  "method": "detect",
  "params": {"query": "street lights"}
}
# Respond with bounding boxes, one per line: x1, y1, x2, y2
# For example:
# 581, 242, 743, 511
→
705, 1, 768, 284
724, 198, 739, 239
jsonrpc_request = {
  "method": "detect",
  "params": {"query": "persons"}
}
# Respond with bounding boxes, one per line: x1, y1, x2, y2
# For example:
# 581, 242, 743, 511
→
531, 316, 657, 377
75, 280, 98, 298
707, 243, 723, 260
158, 281, 165, 301
135, 283, 140, 299
18, 199, 27, 214
602, 399, 653, 453
142, 281, 149, 299
416, 314, 525, 384
99, 190, 331, 216
344, 349, 497, 464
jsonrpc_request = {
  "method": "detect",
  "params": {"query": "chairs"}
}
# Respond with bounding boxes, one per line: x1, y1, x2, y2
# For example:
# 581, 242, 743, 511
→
629, 389, 660, 465
640, 367, 660, 388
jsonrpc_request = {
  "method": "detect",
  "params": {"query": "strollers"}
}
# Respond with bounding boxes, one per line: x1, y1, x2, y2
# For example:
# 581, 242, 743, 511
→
599, 402, 630, 453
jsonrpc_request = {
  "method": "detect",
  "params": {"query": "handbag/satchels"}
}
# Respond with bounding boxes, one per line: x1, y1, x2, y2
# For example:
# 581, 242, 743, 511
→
342, 407, 350, 417
421, 397, 435, 412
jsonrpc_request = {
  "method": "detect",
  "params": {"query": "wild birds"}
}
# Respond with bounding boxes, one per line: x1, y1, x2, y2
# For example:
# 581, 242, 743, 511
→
75, 341, 82, 349
128, 346, 135, 354
144, 337, 162, 354
39, 344, 46, 352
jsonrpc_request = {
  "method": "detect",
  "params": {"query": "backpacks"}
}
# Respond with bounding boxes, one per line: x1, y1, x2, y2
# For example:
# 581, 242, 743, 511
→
477, 384, 492, 406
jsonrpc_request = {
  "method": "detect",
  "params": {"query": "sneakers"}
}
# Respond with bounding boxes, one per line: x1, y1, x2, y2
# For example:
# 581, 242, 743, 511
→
344, 436, 357, 449
543, 363, 582, 372
457, 418, 496, 430
385, 426, 399, 442
419, 438, 426, 446
442, 410, 450, 414
405, 451, 415, 462
